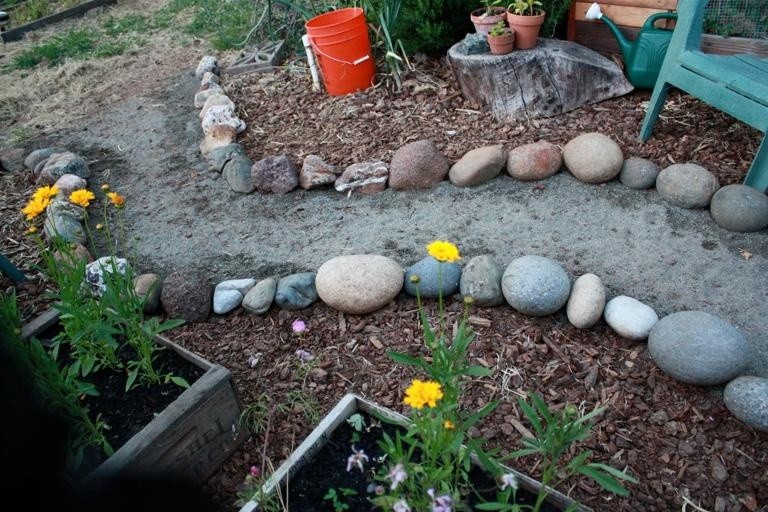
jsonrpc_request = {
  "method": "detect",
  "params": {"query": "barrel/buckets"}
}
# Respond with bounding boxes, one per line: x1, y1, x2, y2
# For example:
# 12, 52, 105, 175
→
305, 8, 376, 96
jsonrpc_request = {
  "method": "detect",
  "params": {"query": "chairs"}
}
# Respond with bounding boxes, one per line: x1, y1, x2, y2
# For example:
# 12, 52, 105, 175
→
636, 0, 768, 195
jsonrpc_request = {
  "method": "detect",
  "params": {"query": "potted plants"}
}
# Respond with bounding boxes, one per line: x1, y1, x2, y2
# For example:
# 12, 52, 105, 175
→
469, 0, 546, 54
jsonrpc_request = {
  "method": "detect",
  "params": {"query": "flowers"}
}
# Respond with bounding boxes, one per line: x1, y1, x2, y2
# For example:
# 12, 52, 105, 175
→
374, 238, 638, 512
17, 181, 193, 392
